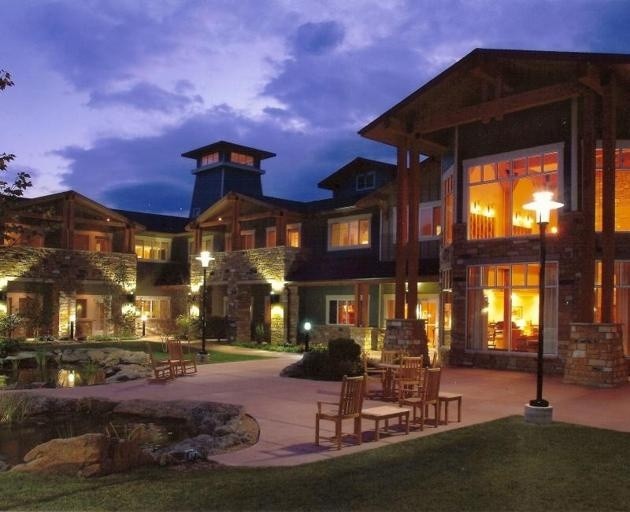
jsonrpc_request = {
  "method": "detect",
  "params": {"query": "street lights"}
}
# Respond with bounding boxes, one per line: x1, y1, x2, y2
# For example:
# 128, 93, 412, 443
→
195, 251, 215, 355
522, 192, 565, 422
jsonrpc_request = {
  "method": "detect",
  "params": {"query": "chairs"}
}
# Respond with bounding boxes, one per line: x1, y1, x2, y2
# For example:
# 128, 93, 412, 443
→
145, 339, 198, 381
314, 348, 464, 451
